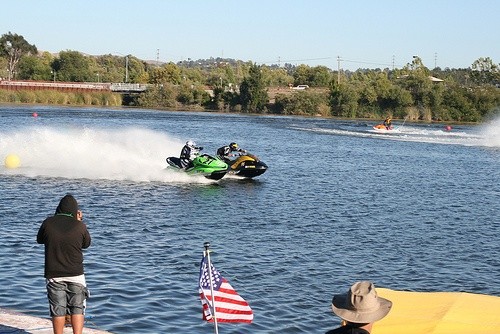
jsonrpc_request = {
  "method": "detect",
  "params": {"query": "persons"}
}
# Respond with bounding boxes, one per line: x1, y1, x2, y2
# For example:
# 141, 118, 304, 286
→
179, 140, 204, 172
217, 142, 249, 167
325, 280, 394, 334
383, 116, 393, 129
36, 194, 93, 333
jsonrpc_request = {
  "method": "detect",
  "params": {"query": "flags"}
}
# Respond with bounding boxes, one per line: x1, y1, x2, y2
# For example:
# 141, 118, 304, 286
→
197, 250, 254, 324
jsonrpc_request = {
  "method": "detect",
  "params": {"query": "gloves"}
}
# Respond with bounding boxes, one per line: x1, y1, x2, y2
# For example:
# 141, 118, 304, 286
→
199, 146, 203, 150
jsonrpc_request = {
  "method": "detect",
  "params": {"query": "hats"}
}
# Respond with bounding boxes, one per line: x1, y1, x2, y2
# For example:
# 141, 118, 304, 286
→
332, 281, 393, 323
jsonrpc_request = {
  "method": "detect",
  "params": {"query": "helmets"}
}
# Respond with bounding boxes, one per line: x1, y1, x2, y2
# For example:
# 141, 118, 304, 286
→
229, 142, 238, 151
186, 140, 196, 150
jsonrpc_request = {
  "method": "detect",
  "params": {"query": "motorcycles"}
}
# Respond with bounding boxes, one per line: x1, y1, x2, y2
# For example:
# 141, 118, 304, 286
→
218, 154, 267, 177
373, 124, 395, 131
166, 154, 230, 181
